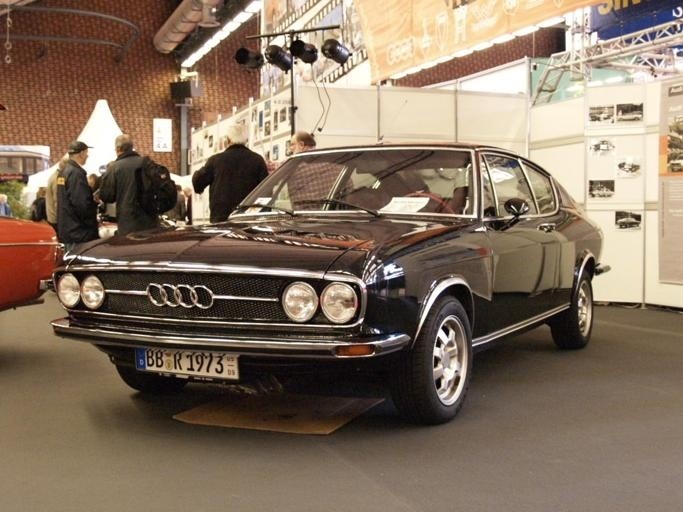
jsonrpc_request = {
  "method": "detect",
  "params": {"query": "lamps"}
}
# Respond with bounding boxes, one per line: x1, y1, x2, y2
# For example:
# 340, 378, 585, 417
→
232, 25, 352, 75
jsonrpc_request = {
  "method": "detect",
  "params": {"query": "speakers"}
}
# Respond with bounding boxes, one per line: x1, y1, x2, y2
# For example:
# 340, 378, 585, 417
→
170, 80, 203, 98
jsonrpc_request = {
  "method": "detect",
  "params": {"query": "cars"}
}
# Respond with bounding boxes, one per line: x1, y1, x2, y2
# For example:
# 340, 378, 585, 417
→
588, 108, 682, 228
0, 215, 67, 313
46, 139, 613, 426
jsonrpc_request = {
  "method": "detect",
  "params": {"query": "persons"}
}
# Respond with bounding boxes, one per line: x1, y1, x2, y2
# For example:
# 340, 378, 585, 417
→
44, 159, 68, 233
191, 123, 270, 224
262, 131, 356, 212
0, 193, 11, 218
30, 172, 192, 228
54, 142, 99, 250
96, 134, 159, 236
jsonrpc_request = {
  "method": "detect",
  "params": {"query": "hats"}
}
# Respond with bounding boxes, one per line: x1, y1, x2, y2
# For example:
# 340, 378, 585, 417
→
65, 141, 94, 154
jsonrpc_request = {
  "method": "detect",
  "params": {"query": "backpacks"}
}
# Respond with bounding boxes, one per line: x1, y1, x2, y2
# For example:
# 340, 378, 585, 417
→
133, 154, 177, 216
